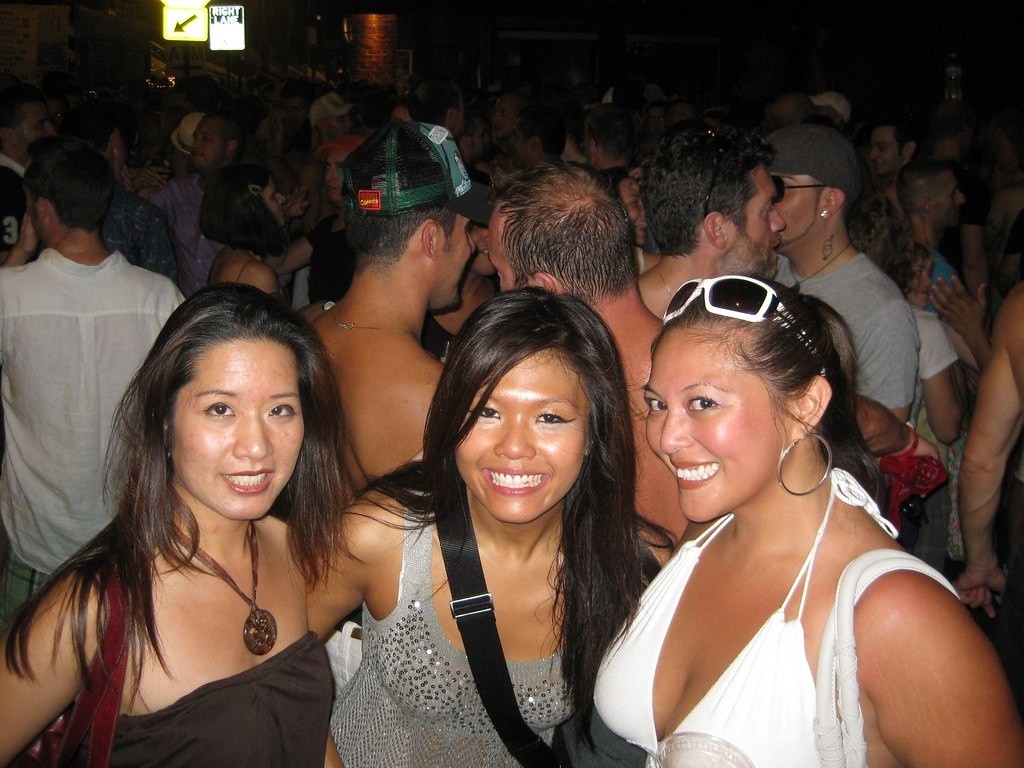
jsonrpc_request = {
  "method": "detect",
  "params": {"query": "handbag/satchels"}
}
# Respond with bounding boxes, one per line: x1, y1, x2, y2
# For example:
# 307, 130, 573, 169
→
5, 560, 130, 768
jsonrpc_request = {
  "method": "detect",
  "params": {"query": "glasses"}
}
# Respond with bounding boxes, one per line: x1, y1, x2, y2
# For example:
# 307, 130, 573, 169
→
662, 275, 826, 378
704, 121, 734, 219
768, 175, 828, 206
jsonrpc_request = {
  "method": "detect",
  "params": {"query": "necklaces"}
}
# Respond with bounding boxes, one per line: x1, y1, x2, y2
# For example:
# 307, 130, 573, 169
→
173, 525, 277, 654
331, 306, 413, 334
791, 242, 852, 289
656, 266, 673, 298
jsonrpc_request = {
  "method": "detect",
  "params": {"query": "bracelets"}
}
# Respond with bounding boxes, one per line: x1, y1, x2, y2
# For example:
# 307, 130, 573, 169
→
890, 426, 918, 459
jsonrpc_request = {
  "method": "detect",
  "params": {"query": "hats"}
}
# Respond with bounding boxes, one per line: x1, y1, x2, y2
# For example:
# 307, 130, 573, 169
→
808, 90, 853, 122
309, 93, 359, 127
315, 134, 368, 161
339, 118, 497, 225
171, 113, 206, 154
765, 123, 861, 204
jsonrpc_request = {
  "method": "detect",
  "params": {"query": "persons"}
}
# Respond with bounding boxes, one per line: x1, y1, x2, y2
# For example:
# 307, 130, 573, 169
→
39, 97, 180, 284
203, 158, 286, 298
484, 162, 688, 542
0, 86, 58, 267
305, 288, 642, 768
154, 110, 247, 299
0, 132, 184, 636
951, 281, 1024, 617
0, 281, 345, 768
23, 71, 1024, 572
296, 119, 495, 506
847, 207, 964, 445
278, 134, 368, 304
591, 276, 1024, 768
637, 116, 939, 489
